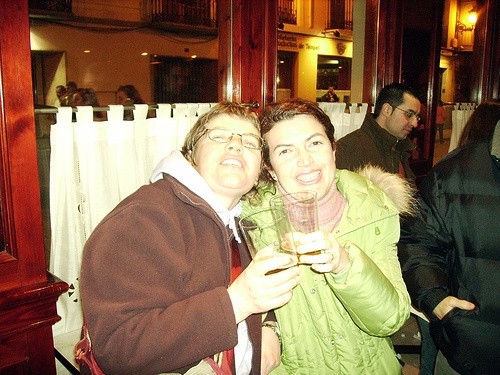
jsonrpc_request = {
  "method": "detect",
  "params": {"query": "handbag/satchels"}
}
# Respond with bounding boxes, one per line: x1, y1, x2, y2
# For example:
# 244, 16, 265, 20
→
73, 324, 227, 375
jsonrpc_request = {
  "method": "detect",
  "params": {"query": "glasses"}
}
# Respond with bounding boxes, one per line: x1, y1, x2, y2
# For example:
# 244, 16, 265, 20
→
391, 105, 421, 122
191, 128, 266, 163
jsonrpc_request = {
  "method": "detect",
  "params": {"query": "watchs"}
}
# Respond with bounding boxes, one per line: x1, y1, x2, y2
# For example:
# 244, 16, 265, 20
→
261, 323, 282, 346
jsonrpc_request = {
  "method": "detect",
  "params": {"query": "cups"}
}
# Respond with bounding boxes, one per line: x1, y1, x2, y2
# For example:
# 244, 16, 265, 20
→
269, 189, 321, 264
239, 208, 299, 275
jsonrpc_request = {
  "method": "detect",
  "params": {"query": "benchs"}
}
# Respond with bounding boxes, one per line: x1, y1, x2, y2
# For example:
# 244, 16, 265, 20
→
51, 119, 440, 375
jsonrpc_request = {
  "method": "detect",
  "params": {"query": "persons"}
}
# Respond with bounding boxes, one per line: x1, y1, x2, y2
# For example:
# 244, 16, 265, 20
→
56, 81, 151, 121
321, 84, 340, 102
397, 98, 500, 375
75, 102, 302, 375
335, 83, 421, 174
238, 97, 411, 375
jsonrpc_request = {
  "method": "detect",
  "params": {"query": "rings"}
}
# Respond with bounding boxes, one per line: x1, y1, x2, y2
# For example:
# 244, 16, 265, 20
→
328, 252, 334, 261
